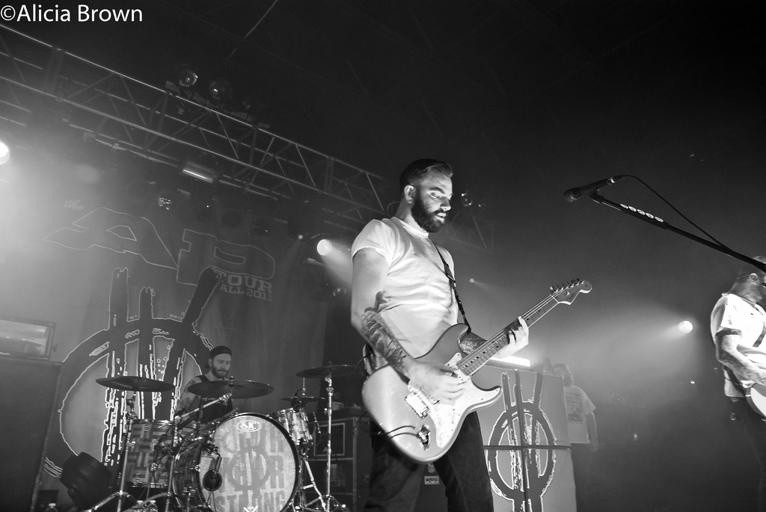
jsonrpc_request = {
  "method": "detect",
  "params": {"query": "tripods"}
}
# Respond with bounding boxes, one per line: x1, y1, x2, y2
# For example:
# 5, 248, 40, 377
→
304, 372, 352, 512
84, 390, 145, 511
126, 420, 201, 512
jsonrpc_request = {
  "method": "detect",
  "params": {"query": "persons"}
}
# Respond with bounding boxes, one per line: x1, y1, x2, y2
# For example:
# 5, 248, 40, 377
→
173, 346, 233, 430
553, 363, 600, 512
350, 158, 530, 512
710, 255, 766, 512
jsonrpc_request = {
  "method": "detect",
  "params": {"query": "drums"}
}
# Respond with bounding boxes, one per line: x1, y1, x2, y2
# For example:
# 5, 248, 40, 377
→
172, 411, 300, 512
123, 418, 196, 490
273, 407, 315, 446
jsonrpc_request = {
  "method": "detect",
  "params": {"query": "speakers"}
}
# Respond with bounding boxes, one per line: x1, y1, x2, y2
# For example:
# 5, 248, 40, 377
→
0, 353, 65, 511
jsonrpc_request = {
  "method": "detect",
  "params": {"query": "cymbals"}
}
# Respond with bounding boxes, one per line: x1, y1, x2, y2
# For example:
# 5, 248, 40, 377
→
281, 395, 326, 402
96, 376, 176, 391
297, 365, 356, 377
188, 381, 275, 398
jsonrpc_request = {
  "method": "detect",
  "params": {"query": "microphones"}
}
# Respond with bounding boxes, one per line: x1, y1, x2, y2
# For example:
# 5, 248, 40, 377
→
562, 174, 623, 203
203, 456, 222, 490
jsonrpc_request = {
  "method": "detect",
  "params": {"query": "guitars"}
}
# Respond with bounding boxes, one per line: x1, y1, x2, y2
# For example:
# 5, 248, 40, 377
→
361, 278, 592, 463
